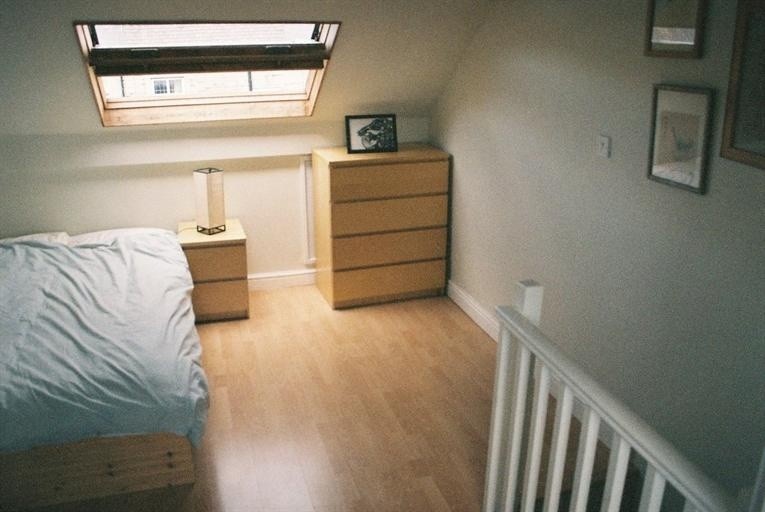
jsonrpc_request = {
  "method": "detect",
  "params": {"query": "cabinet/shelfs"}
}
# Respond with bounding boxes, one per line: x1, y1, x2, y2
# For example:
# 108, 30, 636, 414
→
311, 142, 451, 309
177, 218, 249, 323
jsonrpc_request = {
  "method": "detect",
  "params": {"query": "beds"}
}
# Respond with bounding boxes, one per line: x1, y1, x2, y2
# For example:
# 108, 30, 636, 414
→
0, 226, 210, 511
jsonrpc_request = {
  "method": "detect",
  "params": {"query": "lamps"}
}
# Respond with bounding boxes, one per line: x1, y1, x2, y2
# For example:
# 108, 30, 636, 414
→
193, 167, 226, 236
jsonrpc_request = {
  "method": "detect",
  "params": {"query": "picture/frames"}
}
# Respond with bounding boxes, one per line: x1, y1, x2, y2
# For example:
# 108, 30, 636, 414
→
647, 83, 716, 195
720, 0, 765, 170
345, 112, 398, 154
643, 0, 707, 59
